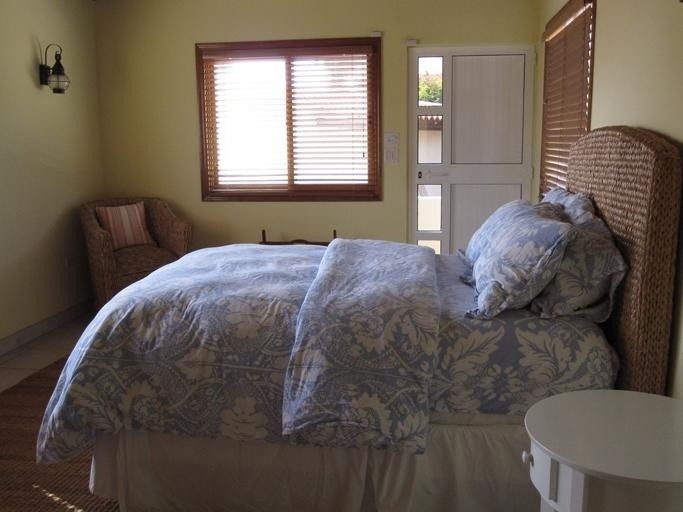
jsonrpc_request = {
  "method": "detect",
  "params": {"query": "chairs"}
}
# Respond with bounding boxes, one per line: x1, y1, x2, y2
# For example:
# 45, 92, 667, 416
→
79, 196, 193, 312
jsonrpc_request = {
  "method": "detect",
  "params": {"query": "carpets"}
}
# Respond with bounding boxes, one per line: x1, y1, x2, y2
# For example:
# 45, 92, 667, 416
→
0, 352, 120, 512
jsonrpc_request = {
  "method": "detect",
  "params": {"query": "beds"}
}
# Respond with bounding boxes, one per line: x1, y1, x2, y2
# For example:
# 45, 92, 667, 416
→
102, 125, 683, 512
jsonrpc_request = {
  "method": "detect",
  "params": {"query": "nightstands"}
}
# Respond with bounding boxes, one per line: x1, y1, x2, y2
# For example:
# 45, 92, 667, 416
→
522, 390, 683, 512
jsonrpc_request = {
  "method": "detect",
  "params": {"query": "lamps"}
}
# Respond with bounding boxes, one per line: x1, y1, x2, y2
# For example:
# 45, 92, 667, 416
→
39, 43, 70, 94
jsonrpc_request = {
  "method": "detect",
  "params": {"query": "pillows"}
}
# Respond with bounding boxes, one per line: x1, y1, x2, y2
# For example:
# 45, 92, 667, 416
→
96, 200, 158, 251
456, 186, 628, 324
